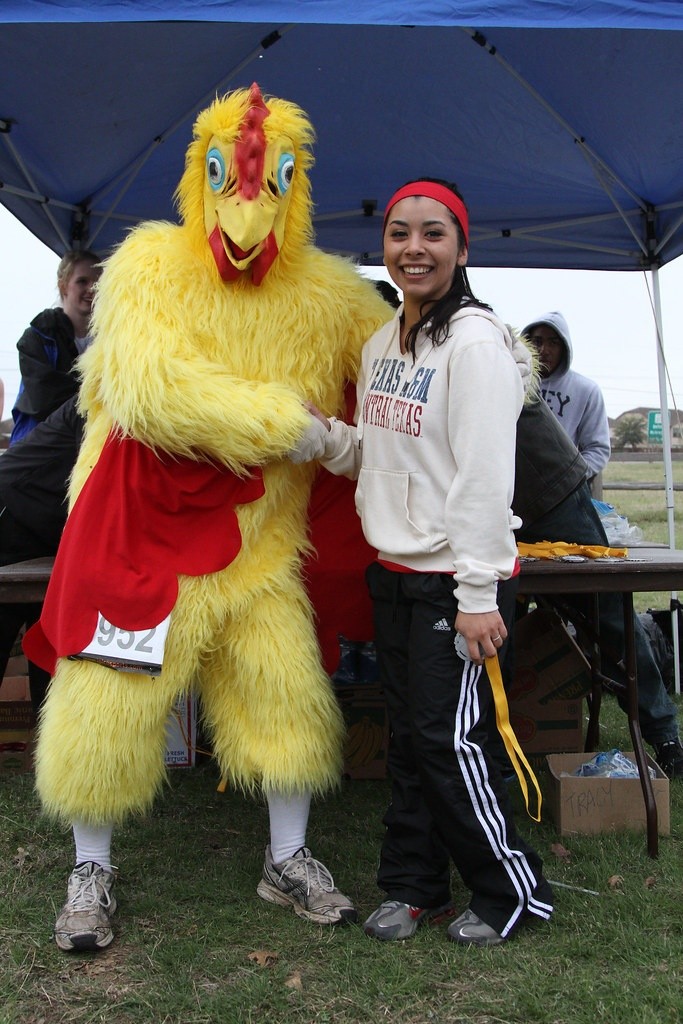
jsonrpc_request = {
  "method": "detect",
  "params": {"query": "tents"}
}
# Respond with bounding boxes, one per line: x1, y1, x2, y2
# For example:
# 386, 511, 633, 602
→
0, 1, 683, 687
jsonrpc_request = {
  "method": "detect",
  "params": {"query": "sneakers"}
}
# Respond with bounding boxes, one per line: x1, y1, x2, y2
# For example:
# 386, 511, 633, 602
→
53, 857, 121, 952
450, 906, 521, 946
257, 845, 354, 928
365, 897, 453, 941
651, 735, 683, 774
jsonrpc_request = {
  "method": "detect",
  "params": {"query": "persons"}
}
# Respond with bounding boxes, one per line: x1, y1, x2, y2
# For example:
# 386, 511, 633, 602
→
31, 88, 357, 944
518, 312, 610, 511
304, 183, 555, 943
7, 253, 106, 458
365, 281, 683, 800
0, 391, 95, 717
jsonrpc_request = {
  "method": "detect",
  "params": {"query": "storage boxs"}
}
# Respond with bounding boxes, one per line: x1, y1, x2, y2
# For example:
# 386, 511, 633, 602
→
0, 654, 39, 776
546, 750, 672, 839
499, 600, 597, 780
327, 682, 390, 783
160, 683, 197, 771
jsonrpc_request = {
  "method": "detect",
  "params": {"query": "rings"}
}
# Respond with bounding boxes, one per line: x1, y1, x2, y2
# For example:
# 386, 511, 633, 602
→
492, 635, 501, 643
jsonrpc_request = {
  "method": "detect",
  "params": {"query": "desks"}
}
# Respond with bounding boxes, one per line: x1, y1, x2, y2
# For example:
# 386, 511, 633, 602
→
0, 548, 683, 858
